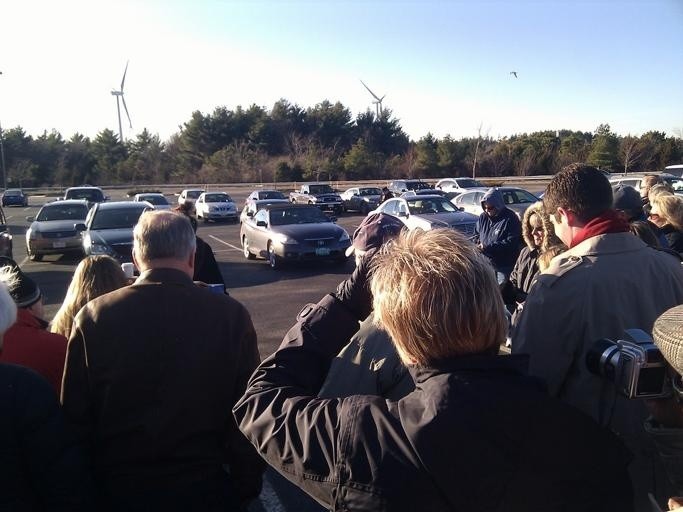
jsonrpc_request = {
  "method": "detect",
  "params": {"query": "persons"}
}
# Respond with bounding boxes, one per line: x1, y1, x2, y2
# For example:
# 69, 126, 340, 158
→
234, 227, 555, 512
477, 163, 682, 511
1, 204, 268, 512
317, 212, 407, 399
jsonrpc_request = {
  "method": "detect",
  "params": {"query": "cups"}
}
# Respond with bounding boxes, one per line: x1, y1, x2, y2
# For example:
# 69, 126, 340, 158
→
207, 282, 225, 298
121, 262, 135, 279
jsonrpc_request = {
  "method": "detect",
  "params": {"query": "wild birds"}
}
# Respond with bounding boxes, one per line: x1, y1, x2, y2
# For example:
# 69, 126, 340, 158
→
510, 71, 518, 78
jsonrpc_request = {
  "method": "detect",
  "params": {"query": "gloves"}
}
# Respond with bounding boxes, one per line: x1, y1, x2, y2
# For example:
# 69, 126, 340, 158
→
331, 246, 389, 322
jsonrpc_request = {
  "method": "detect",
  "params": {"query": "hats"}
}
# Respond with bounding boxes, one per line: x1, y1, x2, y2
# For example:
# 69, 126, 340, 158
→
1, 256, 42, 308
339, 211, 410, 256
613, 184, 645, 212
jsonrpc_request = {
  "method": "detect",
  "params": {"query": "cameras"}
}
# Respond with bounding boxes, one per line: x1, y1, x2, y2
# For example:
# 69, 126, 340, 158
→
585, 327, 676, 401
